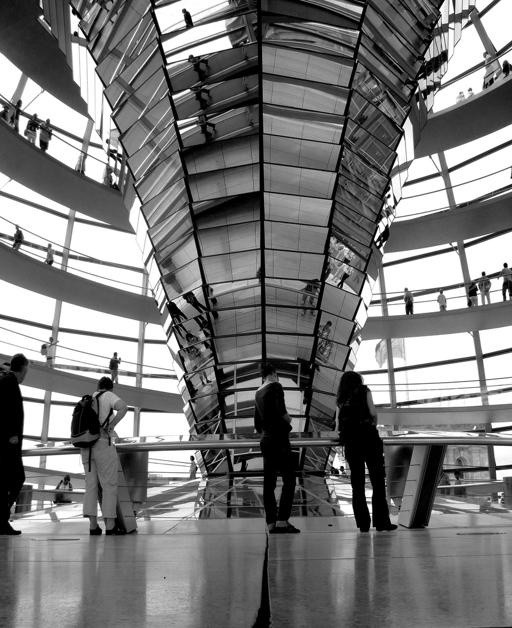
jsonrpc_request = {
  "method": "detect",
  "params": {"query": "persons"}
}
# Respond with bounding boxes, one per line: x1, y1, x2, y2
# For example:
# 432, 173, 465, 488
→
402, 262, 512, 316
413, 48, 448, 103
54, 475, 74, 506
9, 226, 23, 250
340, 465, 347, 478
189, 456, 197, 480
0, 99, 54, 152
44, 337, 60, 368
80, 376, 129, 535
180, 7, 255, 142
109, 352, 121, 384
168, 284, 220, 405
331, 467, 339, 477
0, 353, 31, 536
455, 50, 512, 104
335, 370, 398, 534
439, 458, 467, 499
44, 242, 53, 265
252, 365, 301, 534
297, 184, 396, 362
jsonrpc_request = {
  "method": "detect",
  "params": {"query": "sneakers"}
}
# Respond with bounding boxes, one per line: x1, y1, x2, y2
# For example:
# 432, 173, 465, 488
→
270, 522, 300, 535
105, 525, 125, 536
377, 522, 398, 531
89, 524, 102, 536
359, 525, 370, 532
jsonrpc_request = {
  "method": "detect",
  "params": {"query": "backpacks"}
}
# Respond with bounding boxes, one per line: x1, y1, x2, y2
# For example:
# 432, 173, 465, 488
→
71, 390, 113, 449
